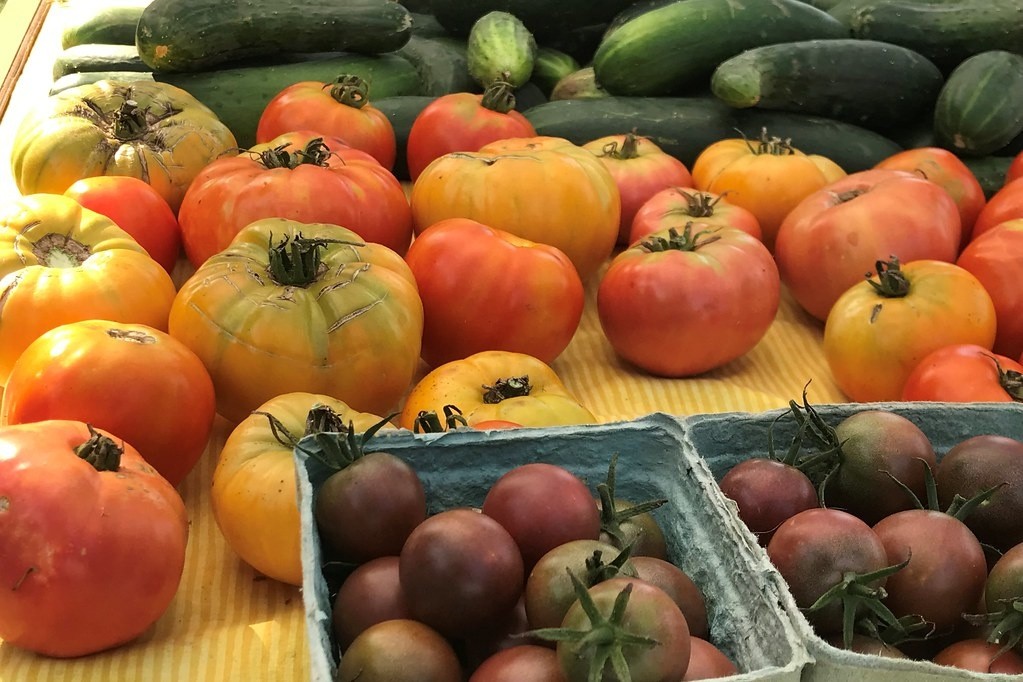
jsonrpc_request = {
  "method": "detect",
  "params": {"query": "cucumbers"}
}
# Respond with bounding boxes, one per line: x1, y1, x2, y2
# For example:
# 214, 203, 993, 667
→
46, 0, 1023, 200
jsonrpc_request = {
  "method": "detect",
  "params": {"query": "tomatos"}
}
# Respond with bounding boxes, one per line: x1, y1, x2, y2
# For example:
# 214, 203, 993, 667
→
0, 80, 1023, 682
